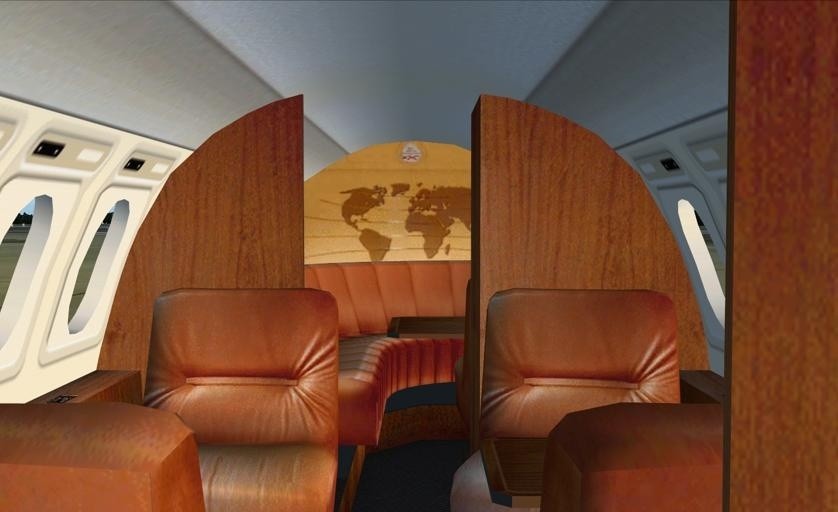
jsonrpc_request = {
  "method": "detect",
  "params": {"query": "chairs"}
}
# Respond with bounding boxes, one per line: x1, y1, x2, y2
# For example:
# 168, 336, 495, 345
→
477, 286, 681, 508
0, 399, 203, 512
145, 287, 341, 512
547, 406, 724, 512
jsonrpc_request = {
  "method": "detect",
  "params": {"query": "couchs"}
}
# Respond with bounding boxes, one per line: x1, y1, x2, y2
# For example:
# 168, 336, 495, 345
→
299, 259, 470, 445
